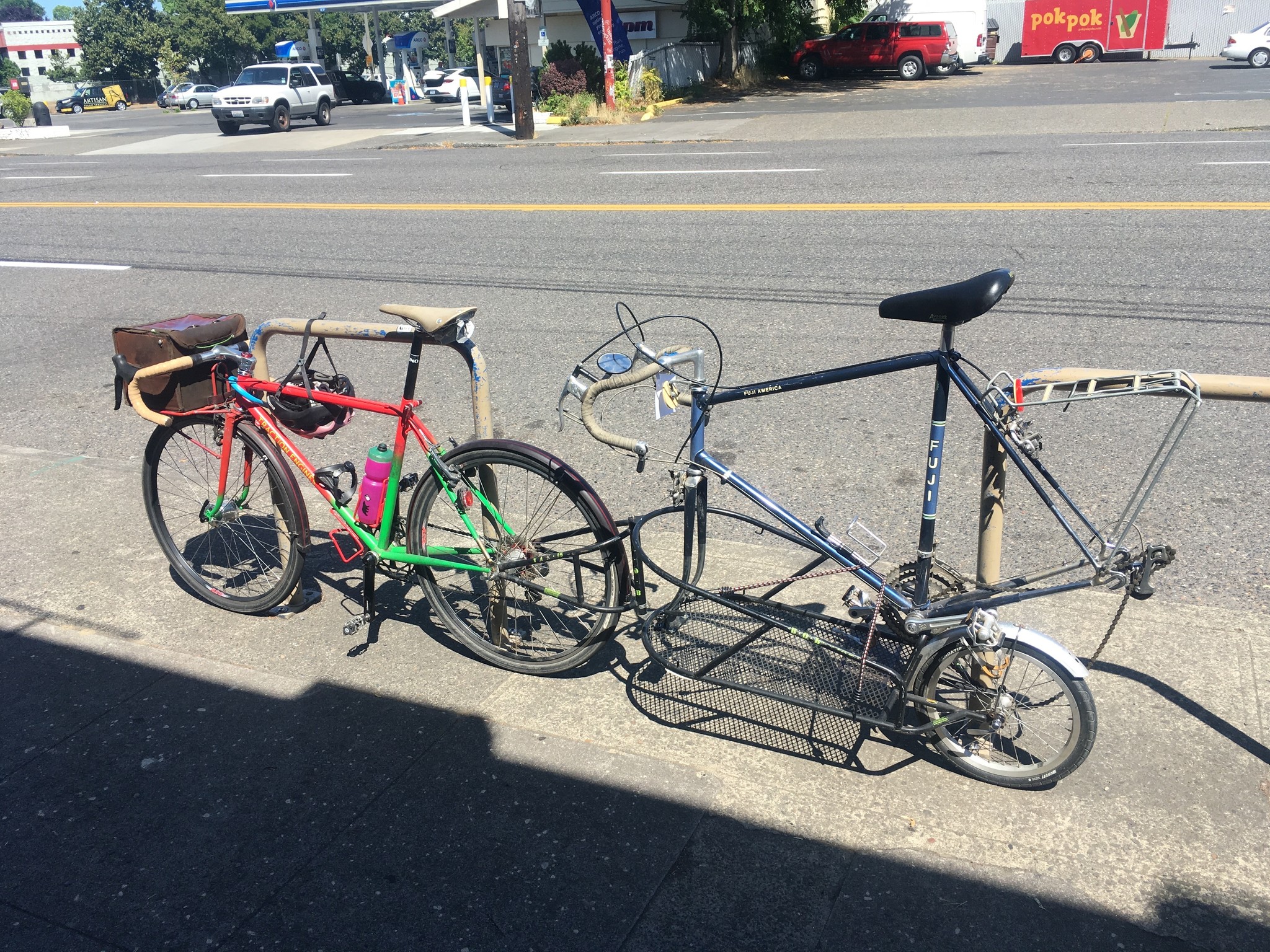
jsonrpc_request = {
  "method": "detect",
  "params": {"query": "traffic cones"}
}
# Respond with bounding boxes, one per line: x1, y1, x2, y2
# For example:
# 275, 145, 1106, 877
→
398, 88, 405, 104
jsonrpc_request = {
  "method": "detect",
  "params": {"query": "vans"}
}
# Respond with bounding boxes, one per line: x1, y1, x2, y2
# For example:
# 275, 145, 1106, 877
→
817, 0, 988, 75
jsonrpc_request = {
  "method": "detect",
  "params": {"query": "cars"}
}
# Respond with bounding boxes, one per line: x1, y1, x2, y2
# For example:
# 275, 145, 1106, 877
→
165, 84, 220, 110
0, 87, 14, 118
356, 74, 396, 90
1221, 20, 1270, 68
157, 82, 195, 108
423, 66, 496, 103
492, 65, 543, 110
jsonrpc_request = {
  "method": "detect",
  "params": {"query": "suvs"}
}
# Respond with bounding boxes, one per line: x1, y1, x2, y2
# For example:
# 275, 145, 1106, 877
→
792, 21, 958, 80
56, 84, 131, 114
211, 61, 338, 134
325, 70, 385, 106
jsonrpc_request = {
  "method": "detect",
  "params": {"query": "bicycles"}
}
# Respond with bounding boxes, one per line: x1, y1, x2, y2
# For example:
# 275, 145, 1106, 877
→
111, 267, 1201, 787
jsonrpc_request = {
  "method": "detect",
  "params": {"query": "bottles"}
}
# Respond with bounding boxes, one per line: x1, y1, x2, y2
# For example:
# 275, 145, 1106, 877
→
358, 443, 393, 525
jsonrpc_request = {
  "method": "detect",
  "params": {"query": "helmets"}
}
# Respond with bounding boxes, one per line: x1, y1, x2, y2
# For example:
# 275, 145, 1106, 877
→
266, 369, 356, 439
